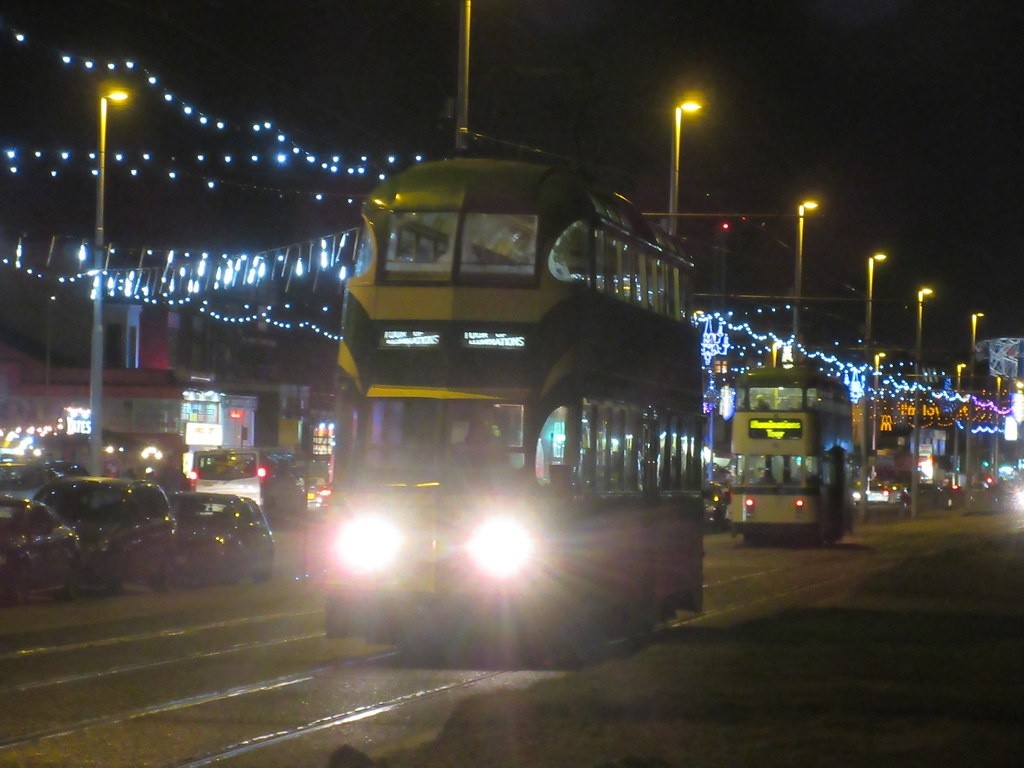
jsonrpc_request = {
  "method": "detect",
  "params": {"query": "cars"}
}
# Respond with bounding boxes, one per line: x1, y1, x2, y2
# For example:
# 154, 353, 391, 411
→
35, 476, 174, 599
230, 498, 276, 582
0, 461, 92, 517
0, 496, 81, 608
306, 476, 330, 515
856, 484, 890, 502
170, 491, 243, 589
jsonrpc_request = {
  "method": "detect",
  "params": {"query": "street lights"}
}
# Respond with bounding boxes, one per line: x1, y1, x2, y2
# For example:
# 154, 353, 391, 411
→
970, 311, 984, 474
793, 198, 816, 368
666, 96, 704, 234
910, 286, 933, 520
89, 85, 133, 477
859, 251, 887, 527
955, 362, 966, 491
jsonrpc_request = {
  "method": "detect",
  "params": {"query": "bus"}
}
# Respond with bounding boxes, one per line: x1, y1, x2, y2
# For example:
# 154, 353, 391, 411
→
190, 449, 270, 523
701, 481, 730, 531
728, 366, 852, 544
317, 153, 707, 659
